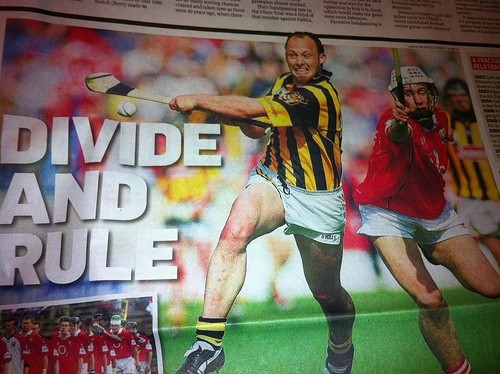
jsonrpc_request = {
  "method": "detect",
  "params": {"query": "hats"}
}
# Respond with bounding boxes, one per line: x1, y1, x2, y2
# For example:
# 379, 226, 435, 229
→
111, 315, 122, 325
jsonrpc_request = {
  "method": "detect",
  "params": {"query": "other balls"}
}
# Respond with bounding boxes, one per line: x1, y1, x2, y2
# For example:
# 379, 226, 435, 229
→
115, 101, 138, 117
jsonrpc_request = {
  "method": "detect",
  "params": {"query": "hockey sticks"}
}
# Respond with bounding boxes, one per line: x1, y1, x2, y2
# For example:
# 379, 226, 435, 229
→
392, 47, 408, 129
85, 72, 273, 130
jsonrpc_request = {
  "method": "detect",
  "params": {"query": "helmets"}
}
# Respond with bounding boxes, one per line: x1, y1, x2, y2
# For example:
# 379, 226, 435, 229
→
443, 77, 468, 95
387, 65, 435, 92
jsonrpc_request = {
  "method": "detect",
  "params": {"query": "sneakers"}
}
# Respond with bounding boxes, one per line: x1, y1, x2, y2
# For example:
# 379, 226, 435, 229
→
325, 358, 353, 374
175, 341, 226, 374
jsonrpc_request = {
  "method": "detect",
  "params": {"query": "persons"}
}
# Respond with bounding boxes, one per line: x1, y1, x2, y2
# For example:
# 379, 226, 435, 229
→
169, 30, 355, 374
0, 314, 153, 374
352, 64, 500, 374
443, 76, 500, 268
